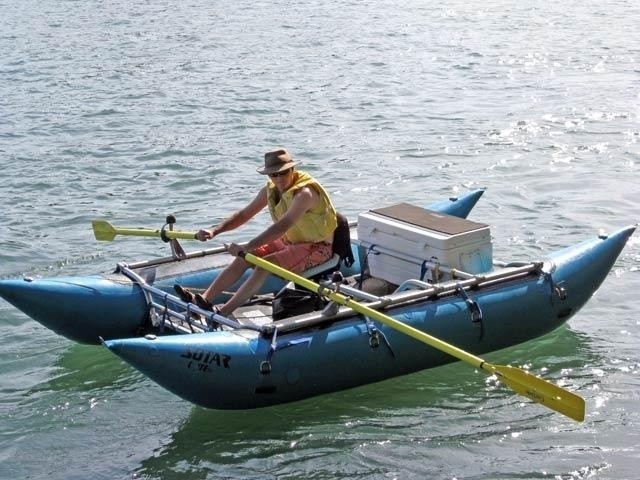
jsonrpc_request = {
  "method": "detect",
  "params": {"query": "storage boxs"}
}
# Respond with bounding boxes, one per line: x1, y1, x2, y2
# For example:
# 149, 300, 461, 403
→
358, 202, 491, 289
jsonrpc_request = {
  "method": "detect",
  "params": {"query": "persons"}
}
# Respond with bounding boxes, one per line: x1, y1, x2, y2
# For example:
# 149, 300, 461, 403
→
173, 148, 339, 330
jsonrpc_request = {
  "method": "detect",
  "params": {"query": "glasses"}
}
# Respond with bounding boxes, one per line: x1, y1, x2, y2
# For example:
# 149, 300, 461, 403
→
268, 169, 290, 178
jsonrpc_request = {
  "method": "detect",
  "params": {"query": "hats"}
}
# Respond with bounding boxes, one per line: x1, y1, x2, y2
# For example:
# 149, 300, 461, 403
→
255, 149, 303, 175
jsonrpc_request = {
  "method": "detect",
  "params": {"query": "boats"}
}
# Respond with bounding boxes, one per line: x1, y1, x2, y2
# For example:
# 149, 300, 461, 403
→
0, 184, 637, 415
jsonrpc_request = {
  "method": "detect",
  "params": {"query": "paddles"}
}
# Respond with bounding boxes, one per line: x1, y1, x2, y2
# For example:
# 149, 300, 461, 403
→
92, 220, 202, 242
224, 244, 584, 423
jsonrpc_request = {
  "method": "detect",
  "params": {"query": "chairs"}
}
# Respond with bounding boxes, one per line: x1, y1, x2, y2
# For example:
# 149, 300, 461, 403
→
292, 207, 349, 313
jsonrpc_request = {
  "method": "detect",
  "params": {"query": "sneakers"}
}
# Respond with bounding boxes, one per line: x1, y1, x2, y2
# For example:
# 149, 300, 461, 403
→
174, 284, 202, 320
195, 293, 228, 330
